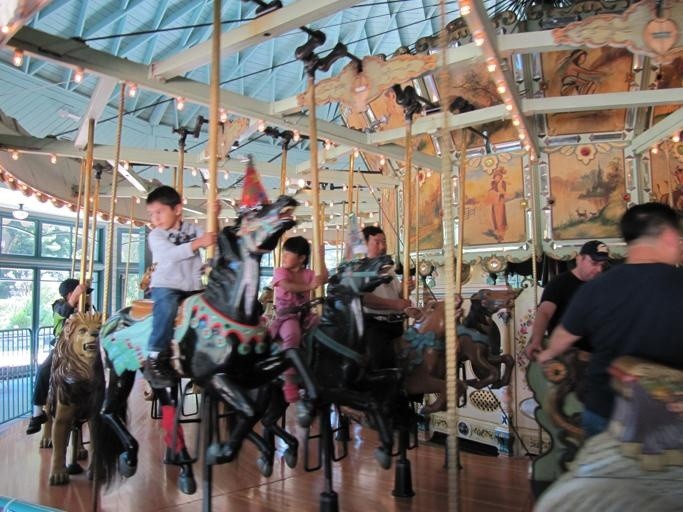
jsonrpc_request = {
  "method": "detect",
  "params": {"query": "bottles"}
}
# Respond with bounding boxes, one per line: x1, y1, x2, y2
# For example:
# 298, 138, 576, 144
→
58, 278, 95, 298
579, 240, 615, 265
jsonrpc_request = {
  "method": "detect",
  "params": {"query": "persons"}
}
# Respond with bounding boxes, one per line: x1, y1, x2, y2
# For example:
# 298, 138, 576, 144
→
524, 239, 616, 361
539, 202, 681, 437
359, 226, 412, 362
524, 203, 682, 372
143, 185, 222, 389
26, 279, 94, 434
270, 236, 329, 403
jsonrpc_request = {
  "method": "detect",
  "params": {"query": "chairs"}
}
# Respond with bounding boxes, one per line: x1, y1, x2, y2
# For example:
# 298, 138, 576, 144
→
26, 410, 48, 435
143, 354, 176, 389
